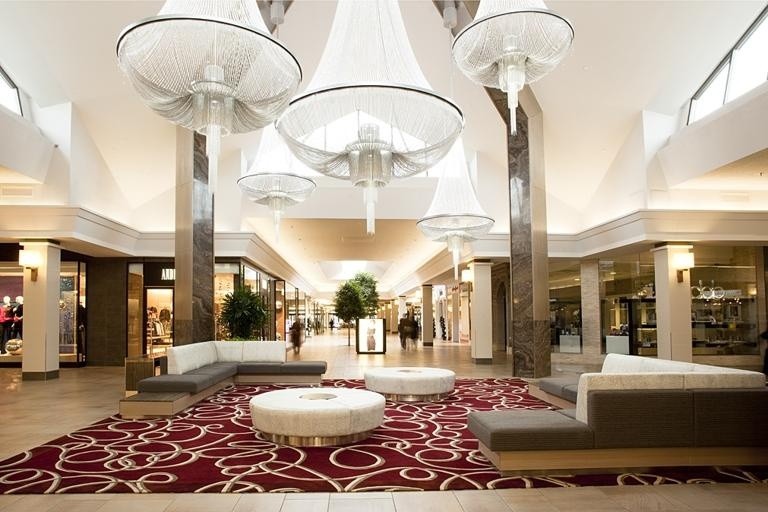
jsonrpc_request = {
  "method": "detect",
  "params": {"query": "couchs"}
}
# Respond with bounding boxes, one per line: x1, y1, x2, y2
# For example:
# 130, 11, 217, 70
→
466, 350, 767, 477
119, 340, 328, 419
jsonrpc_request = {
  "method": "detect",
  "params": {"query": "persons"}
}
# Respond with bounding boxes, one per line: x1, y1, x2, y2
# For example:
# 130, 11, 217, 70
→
759, 328, 768, 383
400, 311, 413, 351
0, 295, 13, 354
366, 320, 376, 350
12, 295, 24, 339
329, 318, 334, 328
292, 316, 302, 354
59, 299, 70, 351
409, 314, 419, 350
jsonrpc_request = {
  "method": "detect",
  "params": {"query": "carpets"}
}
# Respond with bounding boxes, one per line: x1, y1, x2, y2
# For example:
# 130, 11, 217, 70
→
0, 377, 767, 495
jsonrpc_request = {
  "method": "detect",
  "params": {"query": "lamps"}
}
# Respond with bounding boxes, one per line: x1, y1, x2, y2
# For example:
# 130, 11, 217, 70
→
449, 0, 573, 139
19, 249, 41, 280
415, 1, 497, 278
273, 1, 465, 236
113, 2, 303, 196
235, 2, 318, 227
459, 264, 474, 294
676, 253, 695, 283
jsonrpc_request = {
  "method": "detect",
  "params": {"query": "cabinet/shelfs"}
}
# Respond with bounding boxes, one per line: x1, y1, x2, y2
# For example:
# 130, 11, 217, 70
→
622, 298, 755, 354
